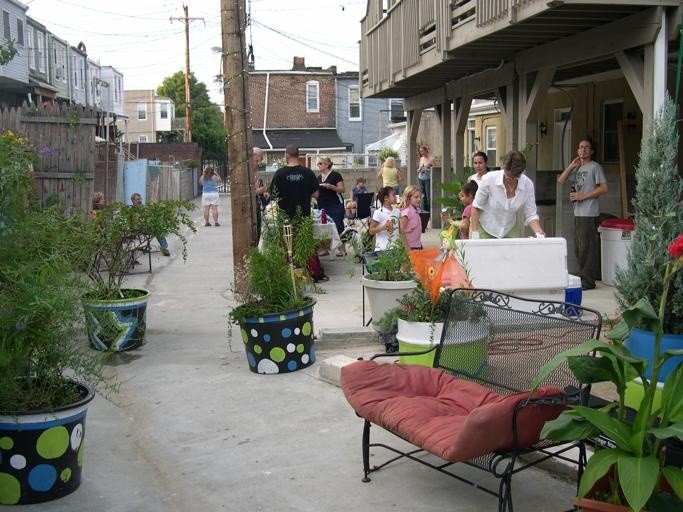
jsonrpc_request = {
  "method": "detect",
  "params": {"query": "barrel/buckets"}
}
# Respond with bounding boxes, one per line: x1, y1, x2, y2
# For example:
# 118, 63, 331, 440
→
396, 315, 492, 385
625, 327, 683, 385
597, 219, 639, 288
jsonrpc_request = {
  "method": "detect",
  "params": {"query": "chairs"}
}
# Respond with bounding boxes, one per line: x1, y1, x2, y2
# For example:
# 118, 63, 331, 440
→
344, 192, 373, 237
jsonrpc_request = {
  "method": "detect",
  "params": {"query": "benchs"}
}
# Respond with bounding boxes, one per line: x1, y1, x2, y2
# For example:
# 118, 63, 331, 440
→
339, 285, 601, 509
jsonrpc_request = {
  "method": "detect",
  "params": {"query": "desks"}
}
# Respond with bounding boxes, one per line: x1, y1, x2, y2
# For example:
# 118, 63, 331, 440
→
258, 203, 343, 261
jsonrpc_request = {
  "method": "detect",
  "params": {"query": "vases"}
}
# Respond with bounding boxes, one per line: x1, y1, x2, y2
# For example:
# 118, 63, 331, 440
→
571, 467, 673, 512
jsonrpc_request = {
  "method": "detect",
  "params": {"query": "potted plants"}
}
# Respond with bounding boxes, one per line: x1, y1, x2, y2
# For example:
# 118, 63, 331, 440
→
65, 192, 201, 357
339, 223, 421, 331
599, 87, 682, 387
0, 130, 134, 506
395, 288, 488, 383
225, 180, 329, 375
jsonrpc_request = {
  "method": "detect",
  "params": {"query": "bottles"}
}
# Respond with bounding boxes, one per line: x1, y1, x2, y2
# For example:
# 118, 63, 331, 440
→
570, 181, 577, 203
320, 209, 327, 224
315, 209, 320, 223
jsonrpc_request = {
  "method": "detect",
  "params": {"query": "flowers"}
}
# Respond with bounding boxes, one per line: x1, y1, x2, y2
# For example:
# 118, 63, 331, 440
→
520, 230, 682, 511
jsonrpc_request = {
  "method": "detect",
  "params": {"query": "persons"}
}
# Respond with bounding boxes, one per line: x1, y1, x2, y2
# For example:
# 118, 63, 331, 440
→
252, 144, 490, 283
469, 151, 546, 240
558, 138, 608, 291
199, 167, 221, 227
92, 192, 106, 220
131, 194, 170, 255
